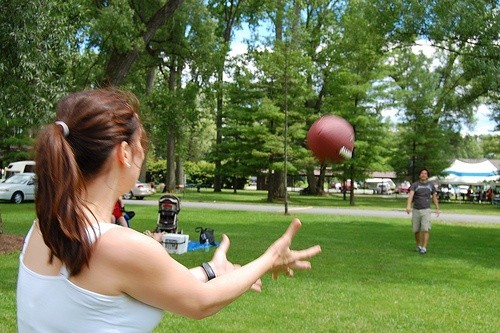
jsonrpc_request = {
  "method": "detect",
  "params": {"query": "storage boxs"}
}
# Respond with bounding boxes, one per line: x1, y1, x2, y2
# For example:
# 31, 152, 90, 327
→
162, 234, 189, 254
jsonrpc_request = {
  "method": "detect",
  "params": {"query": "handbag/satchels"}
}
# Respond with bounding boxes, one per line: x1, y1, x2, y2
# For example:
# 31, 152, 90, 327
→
194, 226, 217, 246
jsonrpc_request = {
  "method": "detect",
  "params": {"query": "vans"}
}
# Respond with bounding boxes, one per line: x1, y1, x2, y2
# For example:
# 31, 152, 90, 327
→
364, 179, 396, 190
4, 161, 37, 181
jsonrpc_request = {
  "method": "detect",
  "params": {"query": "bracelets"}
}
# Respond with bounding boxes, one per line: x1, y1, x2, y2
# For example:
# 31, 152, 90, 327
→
201, 262, 216, 281
436, 207, 440, 210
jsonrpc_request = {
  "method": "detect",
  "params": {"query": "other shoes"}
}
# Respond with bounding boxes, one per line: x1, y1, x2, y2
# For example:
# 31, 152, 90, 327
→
419, 248, 427, 254
416, 246, 421, 252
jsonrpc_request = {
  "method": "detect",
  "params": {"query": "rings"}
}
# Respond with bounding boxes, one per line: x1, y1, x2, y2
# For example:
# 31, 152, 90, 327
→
284, 267, 290, 275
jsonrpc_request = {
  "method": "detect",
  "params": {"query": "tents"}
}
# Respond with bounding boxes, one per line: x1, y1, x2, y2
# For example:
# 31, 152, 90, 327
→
427, 160, 500, 205
441, 184, 474, 196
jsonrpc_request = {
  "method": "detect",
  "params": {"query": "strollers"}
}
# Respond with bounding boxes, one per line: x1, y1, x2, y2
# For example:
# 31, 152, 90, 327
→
153, 195, 183, 235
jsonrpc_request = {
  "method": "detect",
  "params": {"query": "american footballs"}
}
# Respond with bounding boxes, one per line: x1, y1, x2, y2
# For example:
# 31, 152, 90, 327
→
308, 114, 355, 164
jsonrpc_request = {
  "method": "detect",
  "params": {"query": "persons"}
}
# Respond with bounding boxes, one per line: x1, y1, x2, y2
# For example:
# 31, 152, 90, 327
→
112, 196, 128, 227
149, 171, 158, 187
17, 85, 321, 333
466, 186, 500, 204
407, 167, 440, 256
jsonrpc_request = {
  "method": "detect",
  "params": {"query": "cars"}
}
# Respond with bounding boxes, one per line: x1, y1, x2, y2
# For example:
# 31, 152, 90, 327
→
377, 183, 390, 192
335, 179, 358, 190
122, 181, 153, 200
0, 172, 38, 204
399, 182, 411, 193
440, 184, 469, 195
